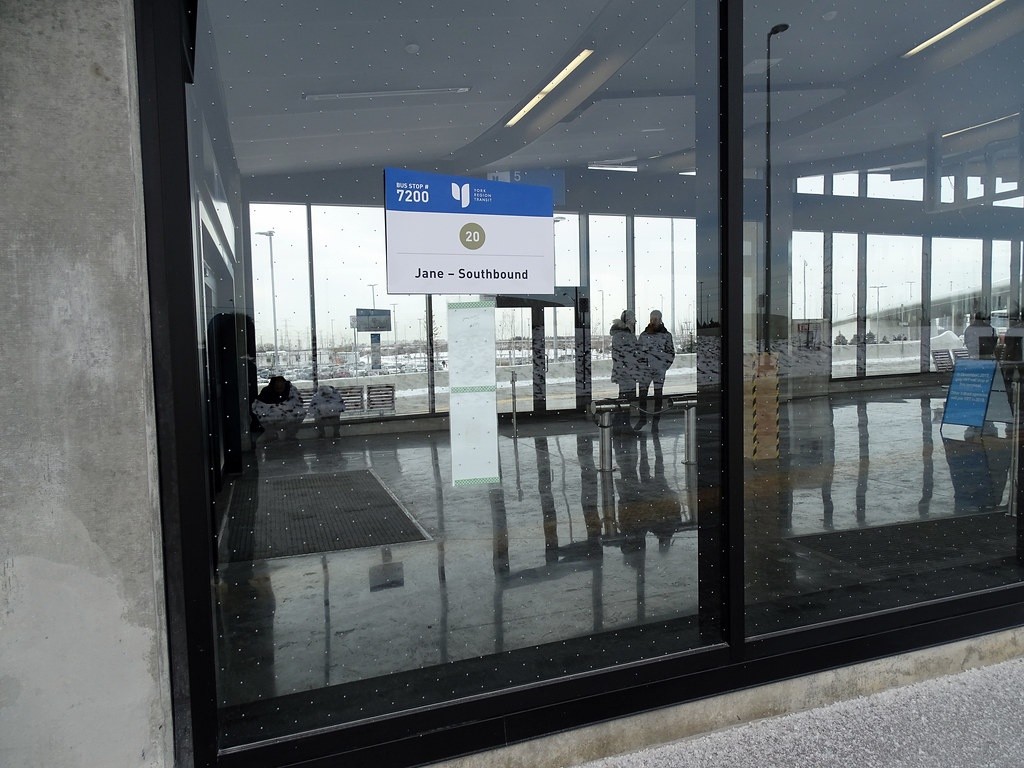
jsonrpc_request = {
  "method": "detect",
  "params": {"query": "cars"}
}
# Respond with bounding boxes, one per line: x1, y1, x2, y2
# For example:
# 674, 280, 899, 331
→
257, 361, 444, 383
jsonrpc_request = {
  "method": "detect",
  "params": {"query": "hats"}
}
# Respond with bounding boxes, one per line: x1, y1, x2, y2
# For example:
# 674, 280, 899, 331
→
621, 310, 634, 322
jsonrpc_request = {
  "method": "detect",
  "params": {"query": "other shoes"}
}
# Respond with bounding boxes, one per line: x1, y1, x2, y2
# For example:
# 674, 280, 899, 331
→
634, 419, 647, 430
614, 423, 635, 438
651, 423, 659, 435
257, 432, 342, 446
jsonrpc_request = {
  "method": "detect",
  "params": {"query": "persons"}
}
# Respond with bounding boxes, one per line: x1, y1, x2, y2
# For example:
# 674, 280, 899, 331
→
1003, 313, 1024, 404
251, 376, 345, 445
609, 310, 675, 434
963, 311, 999, 359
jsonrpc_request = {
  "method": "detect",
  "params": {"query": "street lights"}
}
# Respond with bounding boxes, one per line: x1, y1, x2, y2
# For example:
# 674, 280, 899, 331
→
368, 283, 379, 308
763, 23, 791, 353
390, 304, 399, 344
254, 230, 280, 367
599, 289, 604, 358
906, 281, 916, 307
868, 285, 888, 338
803, 259, 808, 319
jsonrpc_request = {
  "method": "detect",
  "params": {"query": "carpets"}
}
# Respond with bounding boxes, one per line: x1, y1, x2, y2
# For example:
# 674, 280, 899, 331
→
218, 468, 434, 564
